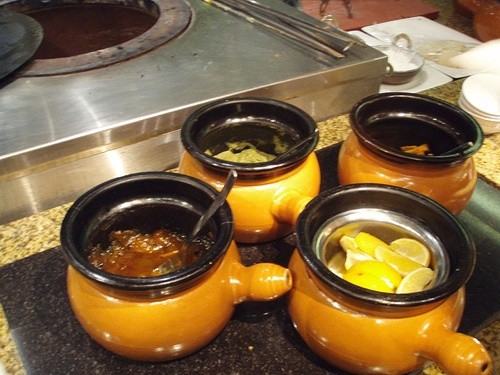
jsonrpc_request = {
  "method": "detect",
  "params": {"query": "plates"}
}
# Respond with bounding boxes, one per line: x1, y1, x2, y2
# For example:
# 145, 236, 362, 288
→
362, 16, 487, 80
346, 30, 454, 94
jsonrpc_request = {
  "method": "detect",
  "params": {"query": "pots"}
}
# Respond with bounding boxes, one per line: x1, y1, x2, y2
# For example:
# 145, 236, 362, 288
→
284, 182, 493, 375
336, 92, 485, 218
178, 97, 321, 244
59, 172, 292, 363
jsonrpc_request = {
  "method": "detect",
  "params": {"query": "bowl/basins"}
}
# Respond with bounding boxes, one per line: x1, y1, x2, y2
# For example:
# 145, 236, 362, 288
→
369, 33, 424, 84
458, 74, 500, 135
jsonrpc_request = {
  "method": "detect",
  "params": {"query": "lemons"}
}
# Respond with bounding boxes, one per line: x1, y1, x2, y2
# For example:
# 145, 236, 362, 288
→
324, 232, 435, 294
